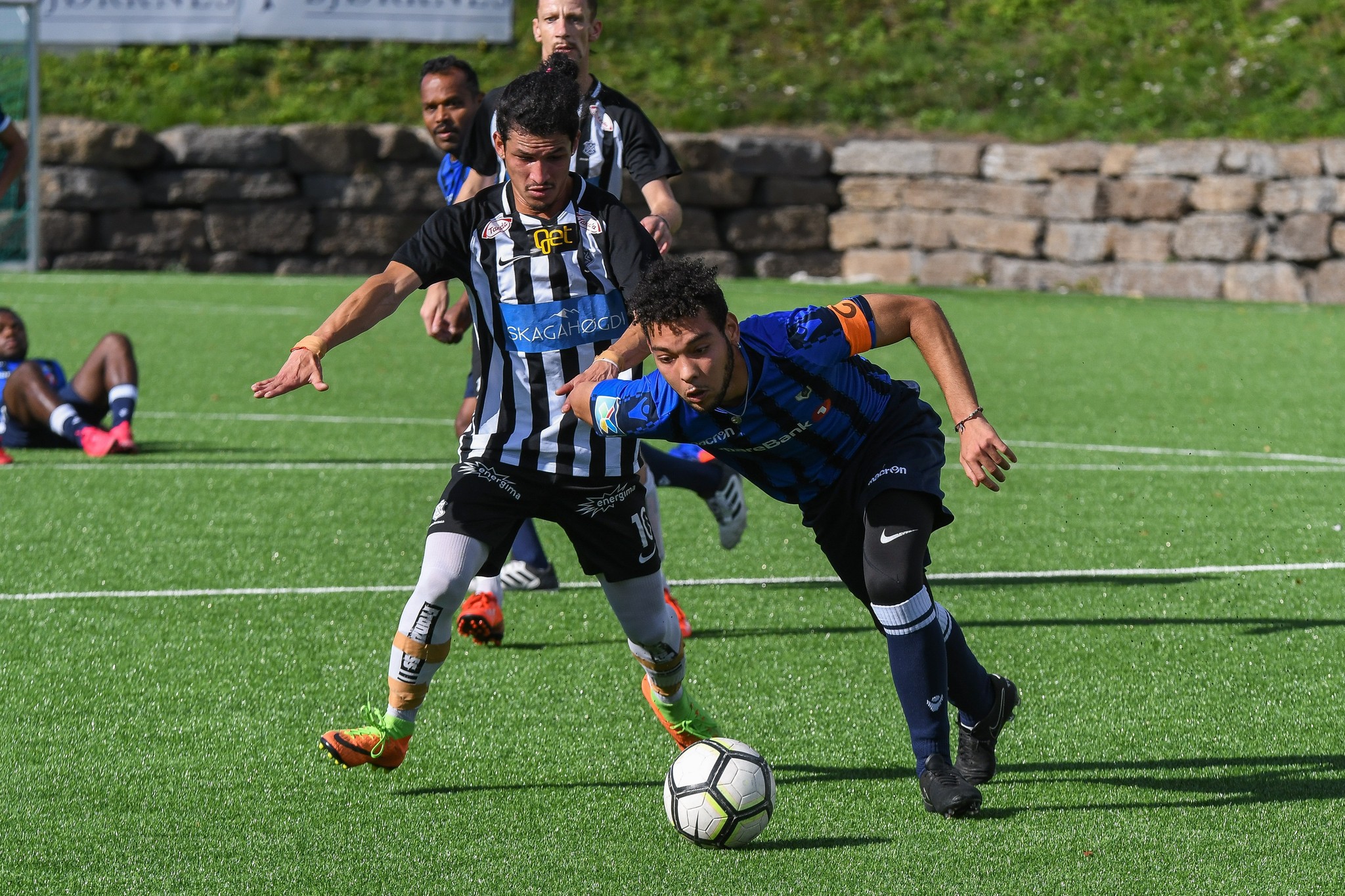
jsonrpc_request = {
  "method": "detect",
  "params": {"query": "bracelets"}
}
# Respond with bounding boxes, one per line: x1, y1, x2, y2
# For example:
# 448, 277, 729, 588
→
592, 357, 620, 370
288, 347, 315, 354
955, 405, 984, 433
649, 213, 671, 230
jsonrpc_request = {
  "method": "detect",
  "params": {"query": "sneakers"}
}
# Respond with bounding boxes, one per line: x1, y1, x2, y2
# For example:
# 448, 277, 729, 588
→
954, 673, 1021, 784
918, 751, 982, 818
110, 420, 135, 449
317, 691, 411, 774
497, 561, 559, 591
703, 457, 747, 550
455, 591, 504, 645
80, 427, 117, 455
641, 673, 723, 749
663, 588, 692, 637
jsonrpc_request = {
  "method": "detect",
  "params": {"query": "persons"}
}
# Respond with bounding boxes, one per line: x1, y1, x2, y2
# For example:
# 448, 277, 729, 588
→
416, 0, 747, 648
0, 102, 140, 463
563, 257, 1021, 813
251, 70, 717, 775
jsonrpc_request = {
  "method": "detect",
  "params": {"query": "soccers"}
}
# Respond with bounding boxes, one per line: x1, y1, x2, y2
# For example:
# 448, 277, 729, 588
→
664, 737, 777, 850
